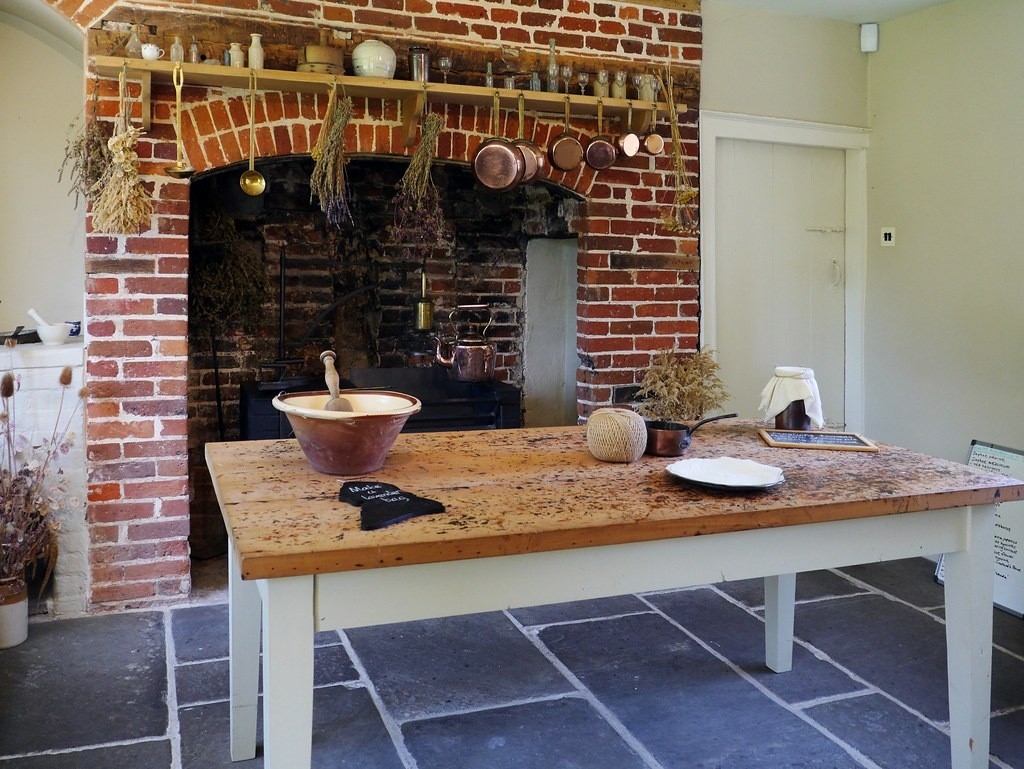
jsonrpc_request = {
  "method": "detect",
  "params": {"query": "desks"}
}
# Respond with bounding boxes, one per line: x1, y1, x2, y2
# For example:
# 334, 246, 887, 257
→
204, 422, 1024, 769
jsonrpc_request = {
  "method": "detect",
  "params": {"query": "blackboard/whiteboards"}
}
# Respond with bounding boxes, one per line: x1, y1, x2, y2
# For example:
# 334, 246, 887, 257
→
935, 439, 1024, 620
758, 428, 879, 452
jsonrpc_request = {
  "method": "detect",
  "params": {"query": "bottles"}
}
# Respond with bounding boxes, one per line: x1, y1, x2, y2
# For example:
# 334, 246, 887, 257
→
124, 25, 142, 59
170, 37, 185, 62
248, 33, 264, 69
486, 61, 493, 87
407, 45, 431, 82
351, 39, 397, 79
529, 70, 541, 92
547, 37, 559, 93
229, 42, 245, 67
188, 42, 199, 64
415, 270, 433, 334
504, 74, 515, 89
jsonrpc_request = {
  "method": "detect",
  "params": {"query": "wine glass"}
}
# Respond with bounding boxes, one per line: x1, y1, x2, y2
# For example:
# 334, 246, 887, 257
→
559, 63, 662, 102
437, 56, 452, 84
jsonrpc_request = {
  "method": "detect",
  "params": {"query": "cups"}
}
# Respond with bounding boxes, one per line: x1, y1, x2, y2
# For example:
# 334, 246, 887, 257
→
140, 43, 165, 60
775, 400, 810, 431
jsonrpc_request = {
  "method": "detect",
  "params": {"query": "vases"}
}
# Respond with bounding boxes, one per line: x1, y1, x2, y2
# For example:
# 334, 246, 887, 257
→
0, 573, 30, 651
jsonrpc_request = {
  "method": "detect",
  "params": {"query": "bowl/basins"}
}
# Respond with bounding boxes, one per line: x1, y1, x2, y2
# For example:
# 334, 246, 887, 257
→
37, 324, 74, 346
271, 388, 422, 476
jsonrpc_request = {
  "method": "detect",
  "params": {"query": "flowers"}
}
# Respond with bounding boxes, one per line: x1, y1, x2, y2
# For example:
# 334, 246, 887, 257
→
0, 365, 90, 594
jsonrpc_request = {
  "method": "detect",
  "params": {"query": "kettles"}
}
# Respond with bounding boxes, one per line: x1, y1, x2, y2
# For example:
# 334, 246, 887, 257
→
434, 304, 497, 383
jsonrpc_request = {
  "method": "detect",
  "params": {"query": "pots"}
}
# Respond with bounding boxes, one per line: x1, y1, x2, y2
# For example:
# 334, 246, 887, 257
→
645, 412, 739, 457
471, 91, 664, 194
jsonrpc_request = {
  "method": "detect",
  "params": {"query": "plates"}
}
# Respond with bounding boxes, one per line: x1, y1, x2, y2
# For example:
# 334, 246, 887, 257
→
667, 469, 784, 495
